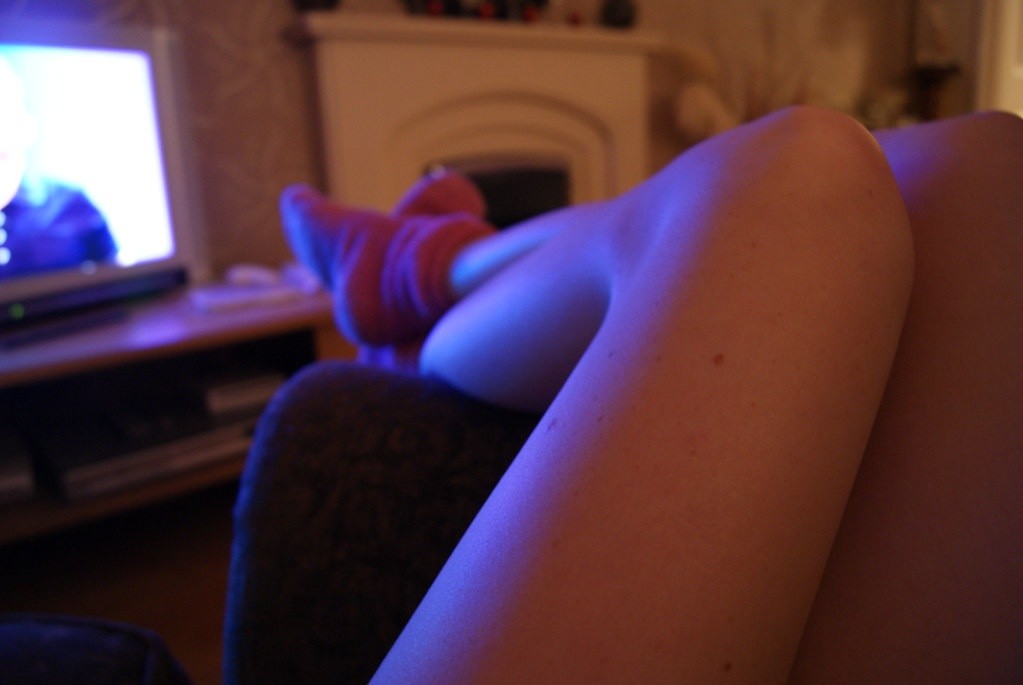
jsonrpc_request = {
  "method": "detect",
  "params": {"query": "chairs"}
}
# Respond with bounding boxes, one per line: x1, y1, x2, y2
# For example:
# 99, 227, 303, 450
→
0, 360, 543, 683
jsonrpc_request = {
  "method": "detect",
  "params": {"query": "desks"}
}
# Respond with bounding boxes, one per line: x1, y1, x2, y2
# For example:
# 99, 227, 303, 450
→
0, 263, 363, 560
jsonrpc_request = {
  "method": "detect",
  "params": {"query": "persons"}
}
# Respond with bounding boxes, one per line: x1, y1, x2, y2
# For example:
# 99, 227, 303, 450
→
279, 102, 1023, 685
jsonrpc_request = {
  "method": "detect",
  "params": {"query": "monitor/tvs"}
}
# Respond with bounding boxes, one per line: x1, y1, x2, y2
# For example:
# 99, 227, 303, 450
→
0, 14, 210, 345
